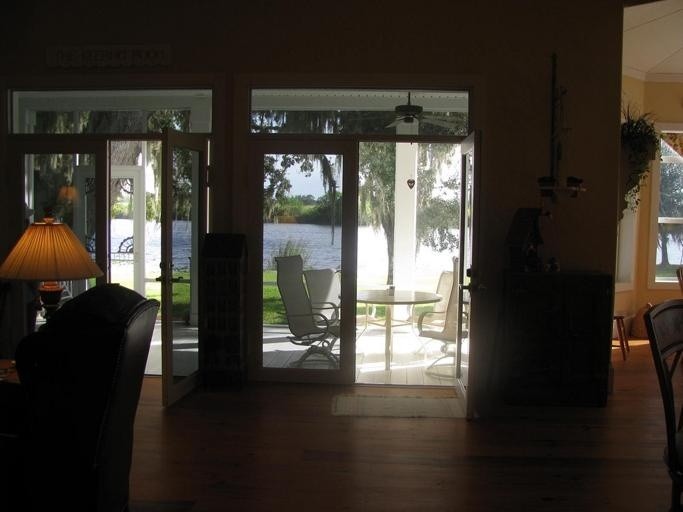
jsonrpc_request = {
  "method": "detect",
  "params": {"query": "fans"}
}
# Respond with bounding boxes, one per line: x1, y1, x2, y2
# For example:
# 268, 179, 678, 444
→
347, 91, 463, 129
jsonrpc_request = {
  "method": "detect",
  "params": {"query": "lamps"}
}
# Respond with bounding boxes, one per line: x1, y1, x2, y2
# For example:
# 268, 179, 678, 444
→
0, 207, 105, 331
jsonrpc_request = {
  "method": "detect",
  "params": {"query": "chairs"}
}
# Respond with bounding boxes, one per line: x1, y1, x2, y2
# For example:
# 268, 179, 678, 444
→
416, 254, 464, 379
1, 285, 162, 511
274, 256, 342, 369
644, 299, 683, 488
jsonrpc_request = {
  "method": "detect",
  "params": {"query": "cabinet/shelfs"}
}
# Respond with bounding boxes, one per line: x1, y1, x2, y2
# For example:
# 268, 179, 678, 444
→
468, 263, 615, 409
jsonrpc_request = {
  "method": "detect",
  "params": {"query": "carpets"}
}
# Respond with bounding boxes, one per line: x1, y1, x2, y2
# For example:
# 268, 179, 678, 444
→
329, 395, 480, 419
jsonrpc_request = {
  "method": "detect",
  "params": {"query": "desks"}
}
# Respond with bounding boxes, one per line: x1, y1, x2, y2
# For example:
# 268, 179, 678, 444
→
339, 290, 443, 384
